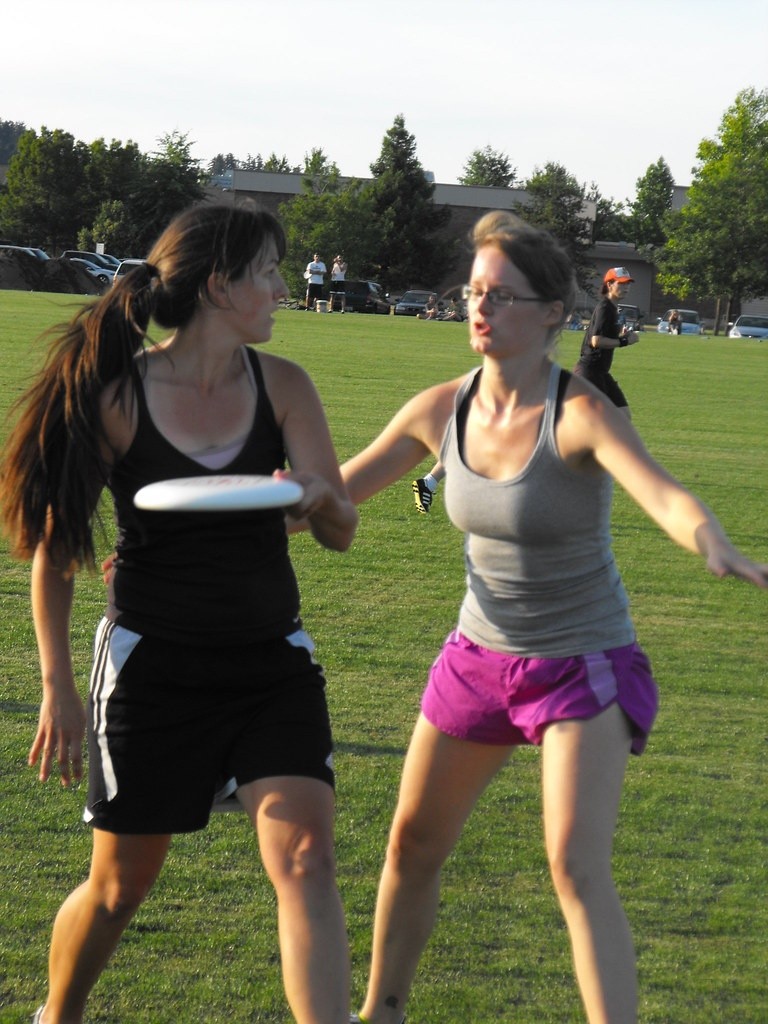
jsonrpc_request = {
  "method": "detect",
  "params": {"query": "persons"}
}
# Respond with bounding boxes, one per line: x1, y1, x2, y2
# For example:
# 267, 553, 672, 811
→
412, 463, 445, 517
0, 202, 359, 1023
101, 203, 768, 1023
573, 266, 640, 421
566, 307, 682, 335
305, 252, 349, 314
417, 295, 465, 322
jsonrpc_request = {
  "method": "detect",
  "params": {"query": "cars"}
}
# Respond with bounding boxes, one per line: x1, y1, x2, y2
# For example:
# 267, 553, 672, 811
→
727, 314, 768, 339
394, 290, 438, 316
618, 302, 645, 330
655, 308, 705, 336
0, 242, 150, 295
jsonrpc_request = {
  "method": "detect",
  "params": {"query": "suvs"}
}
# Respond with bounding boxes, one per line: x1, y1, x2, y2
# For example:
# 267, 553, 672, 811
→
333, 279, 390, 315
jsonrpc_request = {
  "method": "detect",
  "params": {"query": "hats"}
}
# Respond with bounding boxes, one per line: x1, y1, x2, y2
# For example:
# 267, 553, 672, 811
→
604, 267, 635, 283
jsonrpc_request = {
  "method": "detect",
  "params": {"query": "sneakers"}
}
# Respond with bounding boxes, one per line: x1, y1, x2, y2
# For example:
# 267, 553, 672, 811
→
412, 478, 432, 514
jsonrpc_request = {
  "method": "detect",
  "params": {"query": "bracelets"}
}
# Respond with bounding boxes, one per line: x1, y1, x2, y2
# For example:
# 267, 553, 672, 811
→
619, 336, 628, 347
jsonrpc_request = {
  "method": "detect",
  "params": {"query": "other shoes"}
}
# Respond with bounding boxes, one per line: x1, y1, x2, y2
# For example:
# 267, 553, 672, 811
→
305, 307, 309, 311
341, 310, 344, 313
327, 310, 333, 313
314, 307, 317, 311
438, 318, 442, 321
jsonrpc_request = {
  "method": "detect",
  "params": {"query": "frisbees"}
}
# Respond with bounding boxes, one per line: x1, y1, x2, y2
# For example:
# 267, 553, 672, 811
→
132, 474, 305, 514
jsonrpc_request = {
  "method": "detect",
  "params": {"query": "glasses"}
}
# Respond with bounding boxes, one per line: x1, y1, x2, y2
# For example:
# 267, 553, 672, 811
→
461, 283, 549, 312
